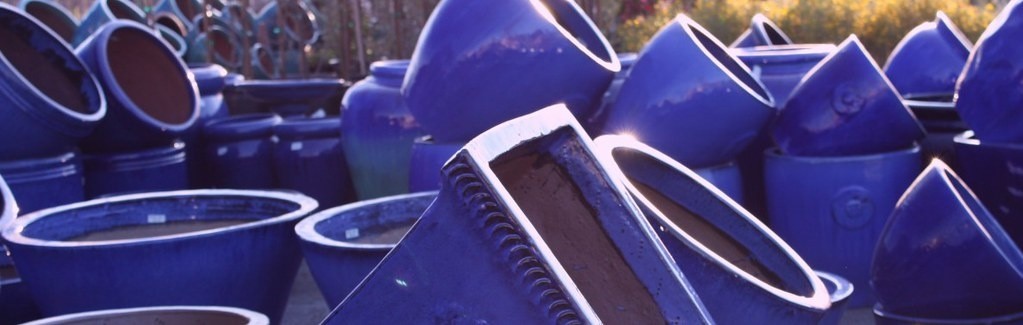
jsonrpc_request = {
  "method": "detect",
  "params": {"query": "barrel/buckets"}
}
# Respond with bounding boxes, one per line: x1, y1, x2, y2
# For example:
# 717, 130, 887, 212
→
186, 0, 322, 79
401, 0, 1023, 325
0, 0, 202, 218
0, 175, 440, 325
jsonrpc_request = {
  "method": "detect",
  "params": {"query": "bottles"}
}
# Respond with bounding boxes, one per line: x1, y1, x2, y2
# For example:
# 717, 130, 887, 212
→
186, 60, 428, 213
